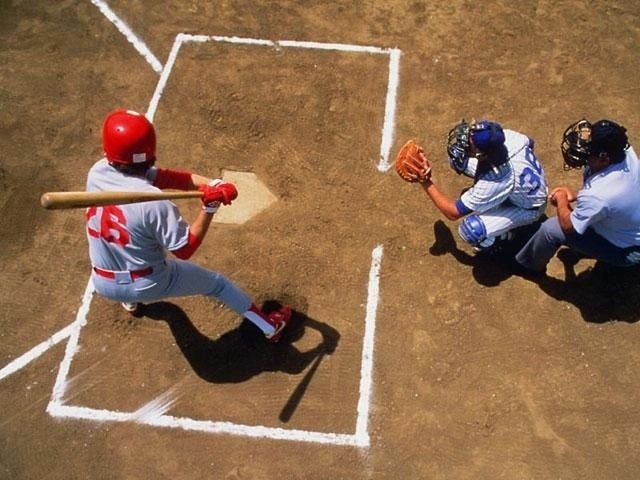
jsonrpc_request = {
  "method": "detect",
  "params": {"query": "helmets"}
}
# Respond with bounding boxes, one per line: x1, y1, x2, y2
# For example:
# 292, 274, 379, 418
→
562, 118, 630, 169
103, 109, 157, 163
447, 121, 505, 175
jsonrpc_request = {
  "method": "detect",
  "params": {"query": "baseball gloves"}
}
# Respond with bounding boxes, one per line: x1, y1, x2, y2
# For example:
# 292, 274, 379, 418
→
395, 140, 432, 183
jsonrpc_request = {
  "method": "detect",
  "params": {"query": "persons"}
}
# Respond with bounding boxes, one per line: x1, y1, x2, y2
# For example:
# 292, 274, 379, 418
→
512, 117, 639, 299
82, 108, 293, 347
395, 117, 551, 258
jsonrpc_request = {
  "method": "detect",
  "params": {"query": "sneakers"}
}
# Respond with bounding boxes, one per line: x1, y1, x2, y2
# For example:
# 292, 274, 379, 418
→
122, 301, 138, 313
472, 234, 515, 255
264, 306, 291, 342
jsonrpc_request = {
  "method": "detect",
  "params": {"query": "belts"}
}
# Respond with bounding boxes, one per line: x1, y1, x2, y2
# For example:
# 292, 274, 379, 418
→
93, 268, 152, 280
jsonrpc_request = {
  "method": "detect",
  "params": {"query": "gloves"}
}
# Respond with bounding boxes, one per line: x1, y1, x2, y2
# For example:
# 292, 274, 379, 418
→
199, 178, 238, 213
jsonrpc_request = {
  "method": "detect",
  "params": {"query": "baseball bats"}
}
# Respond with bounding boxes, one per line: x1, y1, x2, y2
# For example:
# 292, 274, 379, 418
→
40, 191, 204, 210
